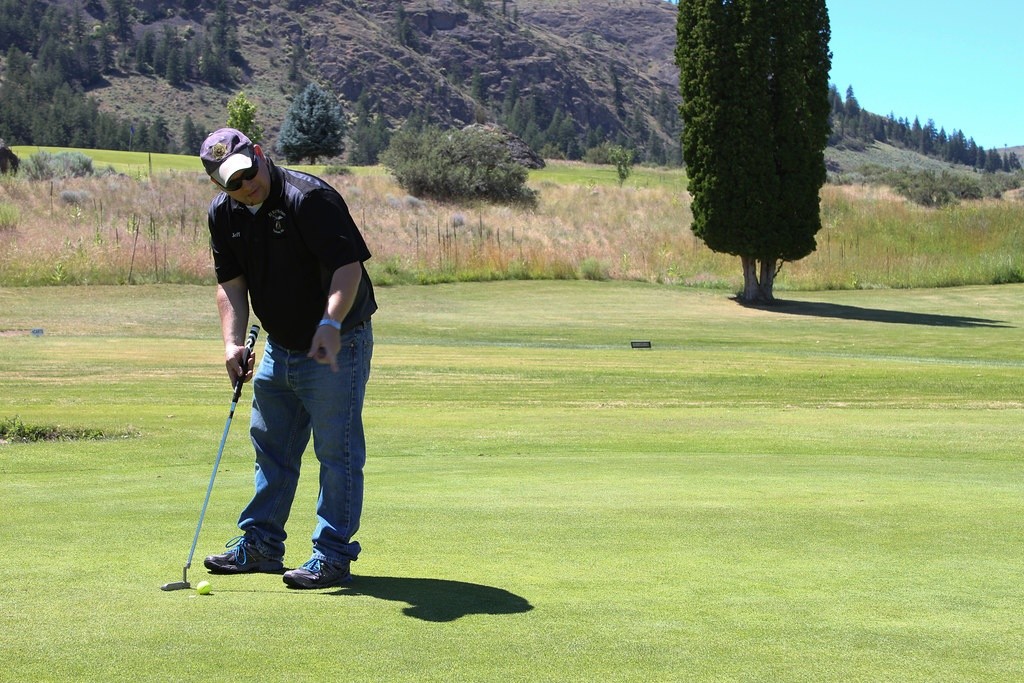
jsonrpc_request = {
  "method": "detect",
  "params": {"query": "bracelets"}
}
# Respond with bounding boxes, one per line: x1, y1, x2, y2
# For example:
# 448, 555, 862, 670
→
318, 319, 341, 330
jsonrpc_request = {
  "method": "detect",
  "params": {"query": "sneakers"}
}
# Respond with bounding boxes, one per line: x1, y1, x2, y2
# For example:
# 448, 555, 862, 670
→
282, 558, 350, 589
204, 532, 283, 573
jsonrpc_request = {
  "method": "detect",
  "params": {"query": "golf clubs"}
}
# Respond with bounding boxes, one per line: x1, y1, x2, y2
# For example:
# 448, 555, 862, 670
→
161, 324, 259, 591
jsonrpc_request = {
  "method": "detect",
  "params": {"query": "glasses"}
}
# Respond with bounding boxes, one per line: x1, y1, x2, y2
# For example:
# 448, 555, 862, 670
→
215, 148, 259, 191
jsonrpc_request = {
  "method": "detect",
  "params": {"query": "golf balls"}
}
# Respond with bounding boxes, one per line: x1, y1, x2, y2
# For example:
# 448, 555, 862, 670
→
197, 580, 212, 595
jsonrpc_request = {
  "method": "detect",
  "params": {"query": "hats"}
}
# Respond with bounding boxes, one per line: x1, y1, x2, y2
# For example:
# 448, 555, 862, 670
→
198, 127, 253, 187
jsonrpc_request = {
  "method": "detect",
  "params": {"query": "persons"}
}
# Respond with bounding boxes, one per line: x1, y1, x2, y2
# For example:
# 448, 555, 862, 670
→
199, 128, 378, 588
